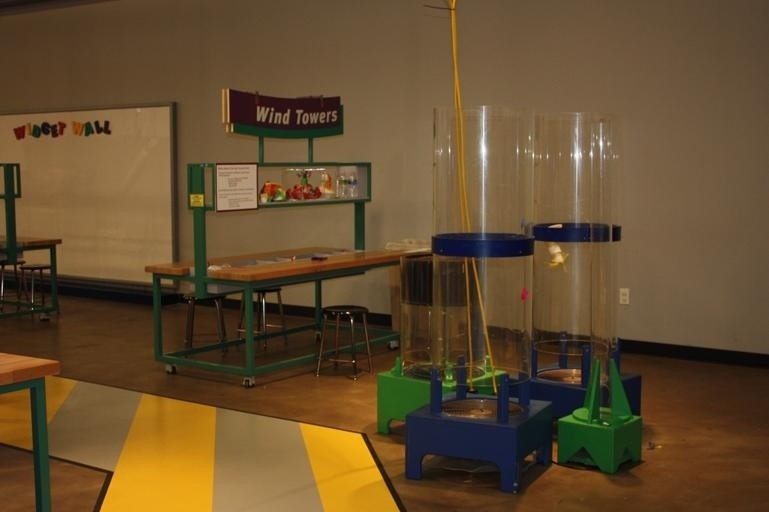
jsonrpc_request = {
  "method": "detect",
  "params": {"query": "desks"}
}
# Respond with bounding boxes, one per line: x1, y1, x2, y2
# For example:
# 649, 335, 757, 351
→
0, 162, 64, 320
1, 352, 60, 512
144, 88, 432, 389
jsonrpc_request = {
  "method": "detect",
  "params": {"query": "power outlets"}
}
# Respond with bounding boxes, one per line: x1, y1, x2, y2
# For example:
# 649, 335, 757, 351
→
619, 288, 630, 305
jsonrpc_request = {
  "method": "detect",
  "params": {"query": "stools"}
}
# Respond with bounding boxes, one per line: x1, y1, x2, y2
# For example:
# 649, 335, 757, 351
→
315, 304, 374, 381
0, 259, 27, 297
236, 286, 288, 351
15, 263, 60, 312
183, 294, 229, 359
